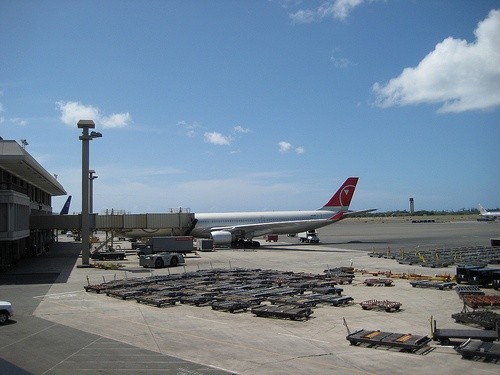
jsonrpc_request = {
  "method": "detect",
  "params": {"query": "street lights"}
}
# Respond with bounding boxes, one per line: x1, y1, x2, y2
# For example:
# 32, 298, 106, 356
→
75, 130, 103, 269
88, 175, 98, 213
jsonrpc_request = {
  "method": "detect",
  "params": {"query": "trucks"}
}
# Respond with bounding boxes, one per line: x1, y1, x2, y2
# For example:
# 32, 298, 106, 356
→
136, 235, 194, 257
298, 229, 320, 244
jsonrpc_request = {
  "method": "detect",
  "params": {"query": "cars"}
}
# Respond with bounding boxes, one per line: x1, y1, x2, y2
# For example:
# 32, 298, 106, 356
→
0, 301, 14, 326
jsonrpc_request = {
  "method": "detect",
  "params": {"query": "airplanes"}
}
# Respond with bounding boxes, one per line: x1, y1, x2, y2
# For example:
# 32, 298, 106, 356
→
122, 177, 378, 248
479, 207, 500, 221
